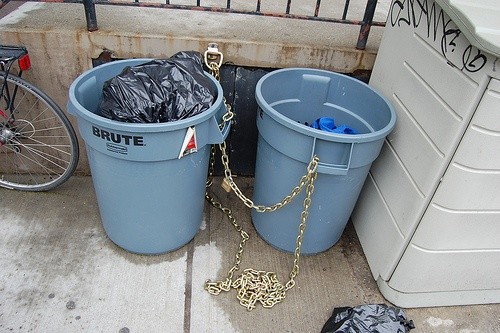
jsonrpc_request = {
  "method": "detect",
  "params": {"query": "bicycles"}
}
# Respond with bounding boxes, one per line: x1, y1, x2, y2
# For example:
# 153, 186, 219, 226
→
0, 44, 79, 193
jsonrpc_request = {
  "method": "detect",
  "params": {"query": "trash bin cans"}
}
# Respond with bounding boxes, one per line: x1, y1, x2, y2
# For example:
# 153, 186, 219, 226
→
65, 56, 236, 256
249, 63, 398, 261
